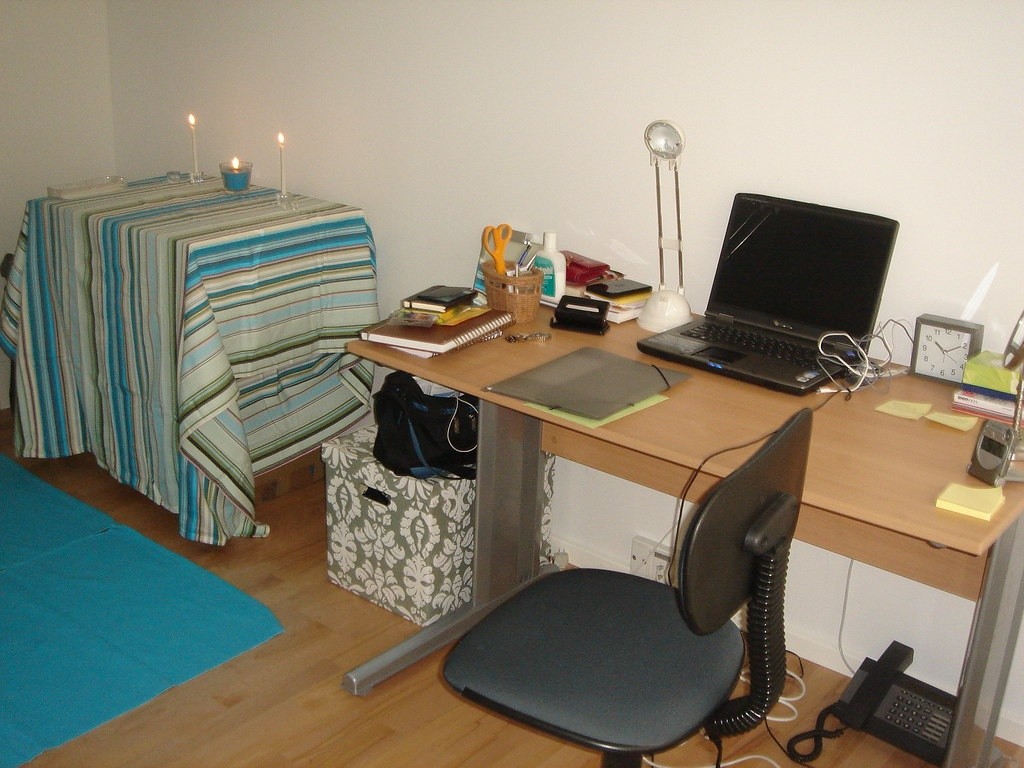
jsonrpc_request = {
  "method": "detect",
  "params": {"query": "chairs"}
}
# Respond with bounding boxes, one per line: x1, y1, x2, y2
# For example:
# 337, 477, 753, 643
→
442, 408, 815, 768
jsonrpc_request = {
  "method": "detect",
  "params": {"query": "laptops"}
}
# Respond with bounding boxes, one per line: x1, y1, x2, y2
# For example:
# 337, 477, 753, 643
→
636, 193, 900, 396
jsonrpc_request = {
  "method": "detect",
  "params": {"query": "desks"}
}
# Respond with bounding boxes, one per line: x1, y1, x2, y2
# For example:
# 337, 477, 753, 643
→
344, 300, 1024, 768
0, 172, 383, 546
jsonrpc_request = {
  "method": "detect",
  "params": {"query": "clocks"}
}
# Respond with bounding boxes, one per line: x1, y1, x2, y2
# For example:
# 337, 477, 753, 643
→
907, 312, 985, 389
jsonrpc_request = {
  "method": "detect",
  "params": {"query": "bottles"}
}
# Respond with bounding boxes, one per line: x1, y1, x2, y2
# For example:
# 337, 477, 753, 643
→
533, 232, 566, 309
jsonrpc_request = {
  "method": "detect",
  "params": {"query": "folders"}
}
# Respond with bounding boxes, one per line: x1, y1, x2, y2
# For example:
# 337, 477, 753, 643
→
480, 347, 693, 421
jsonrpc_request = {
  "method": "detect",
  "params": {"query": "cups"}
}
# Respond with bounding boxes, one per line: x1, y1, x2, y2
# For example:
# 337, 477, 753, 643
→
166, 170, 181, 184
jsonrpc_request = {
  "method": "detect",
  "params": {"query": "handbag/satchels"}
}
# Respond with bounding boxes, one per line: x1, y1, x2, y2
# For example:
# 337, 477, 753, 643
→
559, 250, 611, 283
372, 371, 477, 481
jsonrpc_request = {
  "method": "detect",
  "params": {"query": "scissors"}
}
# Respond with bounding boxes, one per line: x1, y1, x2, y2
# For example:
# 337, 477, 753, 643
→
482, 224, 512, 276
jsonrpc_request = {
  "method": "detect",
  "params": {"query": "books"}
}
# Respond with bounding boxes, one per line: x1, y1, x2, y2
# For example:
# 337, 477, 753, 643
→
355, 286, 517, 360
952, 384, 1024, 429
538, 288, 646, 324
936, 484, 1006, 520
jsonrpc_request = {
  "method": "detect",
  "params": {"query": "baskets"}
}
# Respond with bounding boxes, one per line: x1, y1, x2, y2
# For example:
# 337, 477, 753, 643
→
483, 260, 544, 324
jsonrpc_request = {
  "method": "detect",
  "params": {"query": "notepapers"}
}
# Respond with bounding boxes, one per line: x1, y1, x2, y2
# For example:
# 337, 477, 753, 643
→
935, 484, 1006, 522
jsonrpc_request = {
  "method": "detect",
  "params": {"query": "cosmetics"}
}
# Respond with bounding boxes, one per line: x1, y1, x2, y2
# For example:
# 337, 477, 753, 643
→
534, 231, 566, 299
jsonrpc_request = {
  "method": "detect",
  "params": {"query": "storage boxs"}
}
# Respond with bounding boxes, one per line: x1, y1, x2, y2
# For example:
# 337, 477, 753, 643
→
318, 424, 555, 625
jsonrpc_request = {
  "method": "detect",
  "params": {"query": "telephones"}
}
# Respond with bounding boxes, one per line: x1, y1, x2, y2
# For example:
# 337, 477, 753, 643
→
830, 640, 956, 768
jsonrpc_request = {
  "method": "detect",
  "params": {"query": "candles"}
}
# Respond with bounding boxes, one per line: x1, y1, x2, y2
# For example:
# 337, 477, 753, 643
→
188, 113, 198, 172
277, 132, 287, 195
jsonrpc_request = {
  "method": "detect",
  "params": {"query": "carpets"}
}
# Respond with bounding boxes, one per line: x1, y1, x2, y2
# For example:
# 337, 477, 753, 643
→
0, 453, 288, 768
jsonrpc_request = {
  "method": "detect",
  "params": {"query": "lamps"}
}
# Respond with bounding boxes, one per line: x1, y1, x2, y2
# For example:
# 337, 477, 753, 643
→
637, 119, 692, 334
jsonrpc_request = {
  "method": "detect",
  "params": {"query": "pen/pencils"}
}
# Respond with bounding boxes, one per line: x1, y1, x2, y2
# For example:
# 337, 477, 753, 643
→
518, 244, 533, 266
